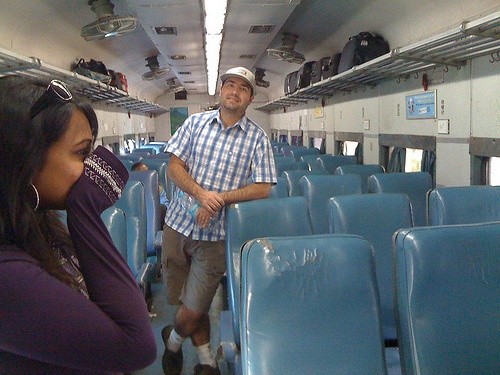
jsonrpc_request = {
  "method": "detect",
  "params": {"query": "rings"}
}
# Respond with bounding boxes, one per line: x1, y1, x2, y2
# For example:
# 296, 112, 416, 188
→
202, 217, 206, 219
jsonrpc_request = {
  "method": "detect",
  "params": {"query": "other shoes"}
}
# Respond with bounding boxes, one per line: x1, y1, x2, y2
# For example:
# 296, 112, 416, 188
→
194, 363, 221, 375
161, 324, 183, 375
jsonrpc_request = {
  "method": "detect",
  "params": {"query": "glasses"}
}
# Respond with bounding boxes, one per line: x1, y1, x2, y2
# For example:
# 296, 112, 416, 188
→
29, 80, 73, 120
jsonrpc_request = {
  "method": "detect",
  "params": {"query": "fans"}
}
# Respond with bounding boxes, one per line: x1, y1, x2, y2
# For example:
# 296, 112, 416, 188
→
166, 77, 184, 95
140, 52, 174, 84
264, 32, 307, 68
78, 0, 140, 44
249, 66, 273, 91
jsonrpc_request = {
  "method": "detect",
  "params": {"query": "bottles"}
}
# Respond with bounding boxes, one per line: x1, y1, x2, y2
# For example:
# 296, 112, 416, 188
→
176, 191, 215, 228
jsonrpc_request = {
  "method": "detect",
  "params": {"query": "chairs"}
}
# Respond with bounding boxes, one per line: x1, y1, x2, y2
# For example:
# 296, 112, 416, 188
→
220, 136, 500, 375
100, 141, 171, 307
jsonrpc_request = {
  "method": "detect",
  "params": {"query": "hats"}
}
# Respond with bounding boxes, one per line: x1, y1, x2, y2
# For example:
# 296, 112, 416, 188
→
220, 66, 256, 97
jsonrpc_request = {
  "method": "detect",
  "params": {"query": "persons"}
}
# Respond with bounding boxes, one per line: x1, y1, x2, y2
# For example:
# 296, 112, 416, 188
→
161, 66, 278, 375
130, 162, 169, 230
0, 74, 157, 375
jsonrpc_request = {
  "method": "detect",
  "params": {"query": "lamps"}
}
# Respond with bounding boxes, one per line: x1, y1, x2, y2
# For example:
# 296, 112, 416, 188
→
199, 0, 230, 97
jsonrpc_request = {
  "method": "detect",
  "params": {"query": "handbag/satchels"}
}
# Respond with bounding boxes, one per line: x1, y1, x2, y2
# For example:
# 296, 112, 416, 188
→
283, 31, 389, 95
76, 58, 128, 93
